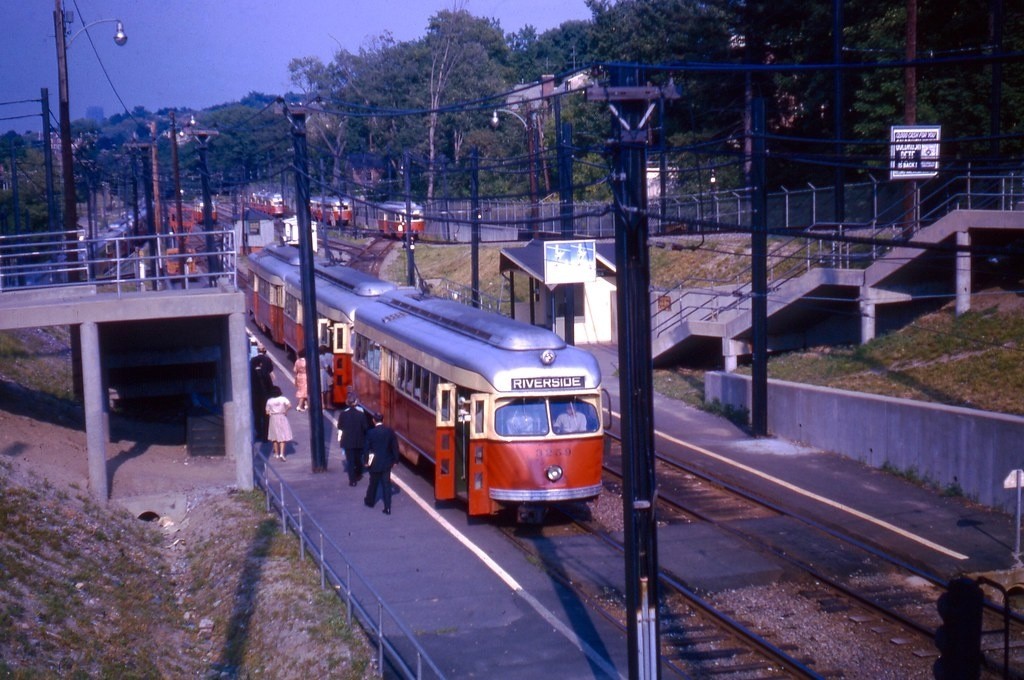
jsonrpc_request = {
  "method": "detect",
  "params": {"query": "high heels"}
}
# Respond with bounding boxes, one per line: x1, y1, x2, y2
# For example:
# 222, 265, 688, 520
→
280, 455, 286, 461
304, 405, 309, 411
296, 406, 305, 412
273, 454, 279, 459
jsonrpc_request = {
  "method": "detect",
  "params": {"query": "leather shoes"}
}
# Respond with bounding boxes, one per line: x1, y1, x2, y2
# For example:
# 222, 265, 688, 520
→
383, 508, 391, 514
364, 497, 373, 507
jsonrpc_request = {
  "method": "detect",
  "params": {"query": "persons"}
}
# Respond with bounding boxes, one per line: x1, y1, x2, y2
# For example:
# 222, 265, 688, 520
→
338, 390, 370, 486
360, 412, 399, 514
293, 345, 334, 412
249, 338, 274, 442
265, 386, 293, 461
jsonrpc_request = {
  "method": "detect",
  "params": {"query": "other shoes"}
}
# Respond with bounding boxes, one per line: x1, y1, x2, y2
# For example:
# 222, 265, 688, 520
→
349, 481, 356, 486
323, 404, 337, 410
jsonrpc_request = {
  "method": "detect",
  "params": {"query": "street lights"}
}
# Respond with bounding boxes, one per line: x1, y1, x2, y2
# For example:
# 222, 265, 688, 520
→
490, 103, 540, 301
150, 121, 183, 281
54, 19, 127, 393
168, 111, 196, 290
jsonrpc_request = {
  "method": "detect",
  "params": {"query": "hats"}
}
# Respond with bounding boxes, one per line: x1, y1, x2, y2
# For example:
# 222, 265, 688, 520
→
346, 396, 358, 406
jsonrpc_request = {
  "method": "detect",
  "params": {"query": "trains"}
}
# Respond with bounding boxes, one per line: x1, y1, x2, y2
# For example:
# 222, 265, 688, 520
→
194, 199, 217, 224
247, 211, 613, 523
310, 196, 352, 224
250, 191, 286, 216
378, 201, 425, 238
